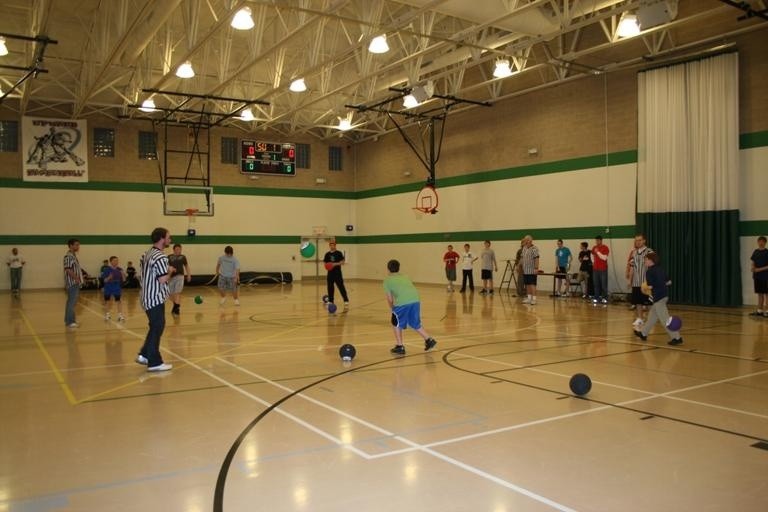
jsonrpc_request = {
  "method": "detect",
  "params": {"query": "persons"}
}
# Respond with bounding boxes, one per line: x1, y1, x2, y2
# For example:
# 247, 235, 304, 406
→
749, 235, 768, 319
100, 255, 127, 323
215, 245, 242, 307
166, 243, 192, 315
167, 312, 192, 370
631, 252, 685, 346
62, 239, 82, 329
323, 242, 350, 306
479, 293, 497, 336
64, 326, 84, 398
512, 231, 658, 326
99, 260, 110, 291
479, 240, 497, 295
460, 291, 474, 334
80, 268, 88, 279
513, 298, 679, 359
381, 258, 437, 356
752, 320, 768, 367
5, 248, 26, 297
442, 244, 460, 292
9, 294, 24, 337
444, 290, 457, 335
320, 305, 349, 356
217, 304, 242, 365
124, 260, 138, 287
459, 244, 479, 293
134, 228, 177, 371
103, 321, 125, 386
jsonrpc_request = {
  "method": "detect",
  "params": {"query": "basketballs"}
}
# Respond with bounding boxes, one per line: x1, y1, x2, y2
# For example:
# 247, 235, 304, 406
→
339, 344, 357, 360
665, 315, 683, 331
327, 303, 337, 314
570, 373, 592, 396
632, 317, 646, 327
642, 280, 653, 295
194, 294, 204, 304
321, 294, 330, 304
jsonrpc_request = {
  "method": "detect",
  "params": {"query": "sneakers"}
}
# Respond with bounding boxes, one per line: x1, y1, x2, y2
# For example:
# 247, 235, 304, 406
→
147, 362, 173, 372
424, 337, 437, 352
667, 337, 684, 346
581, 294, 608, 304
219, 296, 228, 305
522, 298, 537, 305
116, 313, 124, 321
135, 354, 148, 365
233, 298, 240, 306
633, 329, 647, 341
64, 319, 80, 328
104, 312, 112, 321
389, 344, 405, 355
628, 304, 636, 311
749, 311, 768, 317
447, 285, 494, 294
642, 304, 649, 311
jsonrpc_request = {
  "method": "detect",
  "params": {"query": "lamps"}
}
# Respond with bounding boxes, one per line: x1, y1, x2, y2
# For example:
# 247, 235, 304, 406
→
0, 33, 9, 56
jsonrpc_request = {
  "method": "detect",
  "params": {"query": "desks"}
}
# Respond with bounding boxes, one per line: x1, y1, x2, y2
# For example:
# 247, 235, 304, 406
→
537, 272, 578, 297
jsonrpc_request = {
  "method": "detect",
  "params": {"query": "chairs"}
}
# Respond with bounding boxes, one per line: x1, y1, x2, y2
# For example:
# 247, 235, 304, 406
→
563, 273, 586, 298
